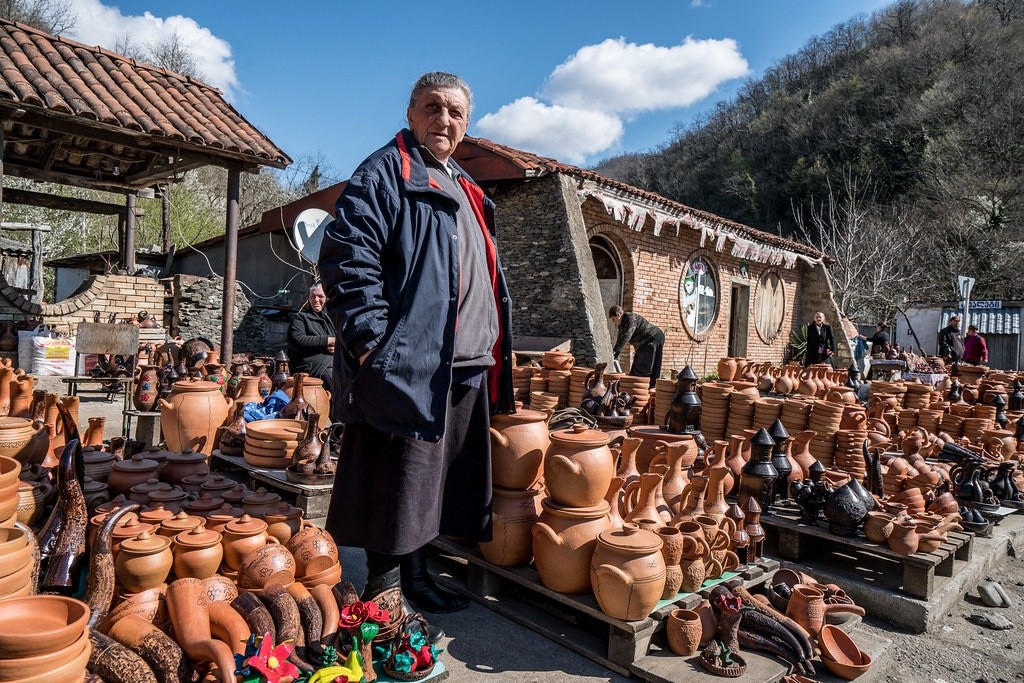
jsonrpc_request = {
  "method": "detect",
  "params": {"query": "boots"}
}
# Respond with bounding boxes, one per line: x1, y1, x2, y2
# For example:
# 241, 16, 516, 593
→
367, 563, 445, 643
399, 547, 470, 614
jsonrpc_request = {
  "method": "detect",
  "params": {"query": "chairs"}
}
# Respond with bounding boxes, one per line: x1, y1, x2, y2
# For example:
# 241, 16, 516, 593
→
62, 321, 140, 439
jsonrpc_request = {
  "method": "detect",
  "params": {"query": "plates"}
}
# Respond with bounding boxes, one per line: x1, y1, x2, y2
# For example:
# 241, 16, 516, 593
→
512, 348, 1024, 553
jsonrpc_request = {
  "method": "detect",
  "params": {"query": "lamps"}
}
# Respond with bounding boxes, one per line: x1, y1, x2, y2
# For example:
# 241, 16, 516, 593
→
113, 162, 120, 176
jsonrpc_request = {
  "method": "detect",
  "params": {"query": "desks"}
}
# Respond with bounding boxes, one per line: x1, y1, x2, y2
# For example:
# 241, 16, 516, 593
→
211, 450, 336, 519
870, 358, 906, 383
762, 514, 975, 600
123, 410, 164, 448
425, 536, 778, 677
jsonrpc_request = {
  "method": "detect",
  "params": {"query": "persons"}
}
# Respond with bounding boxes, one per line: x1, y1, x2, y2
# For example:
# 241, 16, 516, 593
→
939, 315, 964, 376
964, 324, 988, 366
317, 71, 518, 618
804, 311, 836, 368
287, 283, 336, 391
608, 305, 666, 390
858, 321, 890, 380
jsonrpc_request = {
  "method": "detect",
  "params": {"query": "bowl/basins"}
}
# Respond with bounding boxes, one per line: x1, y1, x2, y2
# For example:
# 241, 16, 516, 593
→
817, 624, 862, 665
0, 350, 342, 683
667, 609, 703, 656
820, 651, 872, 681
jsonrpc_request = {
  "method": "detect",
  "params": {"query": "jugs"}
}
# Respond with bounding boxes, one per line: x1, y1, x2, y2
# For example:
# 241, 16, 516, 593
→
478, 399, 740, 622
785, 584, 866, 637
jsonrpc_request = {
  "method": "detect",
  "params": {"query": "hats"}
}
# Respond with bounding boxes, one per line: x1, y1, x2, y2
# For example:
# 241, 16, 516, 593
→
950, 316, 959, 321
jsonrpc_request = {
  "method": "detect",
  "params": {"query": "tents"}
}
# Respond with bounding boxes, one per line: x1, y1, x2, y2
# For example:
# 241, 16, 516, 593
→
1, 17, 294, 373
